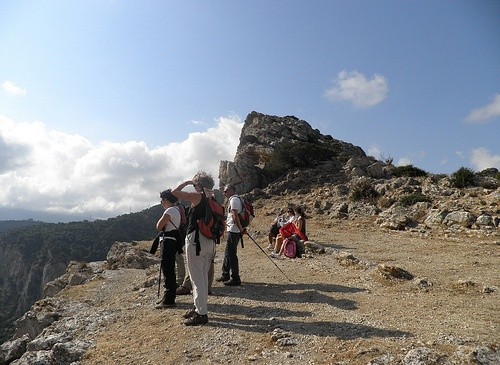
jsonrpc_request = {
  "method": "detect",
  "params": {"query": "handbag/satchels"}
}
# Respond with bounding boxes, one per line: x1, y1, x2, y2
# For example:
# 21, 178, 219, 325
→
280, 223, 295, 238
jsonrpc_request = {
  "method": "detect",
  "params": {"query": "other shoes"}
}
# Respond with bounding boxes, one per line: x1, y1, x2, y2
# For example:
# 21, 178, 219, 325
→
183, 307, 196, 317
156, 296, 164, 304
184, 313, 208, 326
155, 302, 175, 308
176, 285, 190, 295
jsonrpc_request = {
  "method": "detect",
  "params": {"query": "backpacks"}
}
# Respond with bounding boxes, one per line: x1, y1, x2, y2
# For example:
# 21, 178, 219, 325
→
191, 192, 225, 244
165, 204, 187, 247
229, 196, 255, 227
284, 239, 296, 258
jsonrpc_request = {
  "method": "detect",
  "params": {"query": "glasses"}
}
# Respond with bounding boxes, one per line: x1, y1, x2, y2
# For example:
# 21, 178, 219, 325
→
287, 211, 293, 213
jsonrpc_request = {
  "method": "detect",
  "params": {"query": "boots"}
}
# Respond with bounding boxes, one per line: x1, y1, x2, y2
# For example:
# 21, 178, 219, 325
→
215, 273, 230, 282
223, 275, 241, 286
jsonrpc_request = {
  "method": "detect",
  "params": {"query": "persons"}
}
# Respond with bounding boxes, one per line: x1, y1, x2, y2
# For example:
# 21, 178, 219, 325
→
269, 208, 296, 257
173, 198, 186, 290
271, 207, 306, 258
267, 210, 290, 250
156, 190, 186, 310
171, 171, 226, 326
216, 183, 248, 286
175, 188, 217, 294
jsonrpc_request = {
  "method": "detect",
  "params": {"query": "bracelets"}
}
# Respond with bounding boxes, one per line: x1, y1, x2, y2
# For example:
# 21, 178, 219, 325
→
185, 181, 188, 185
